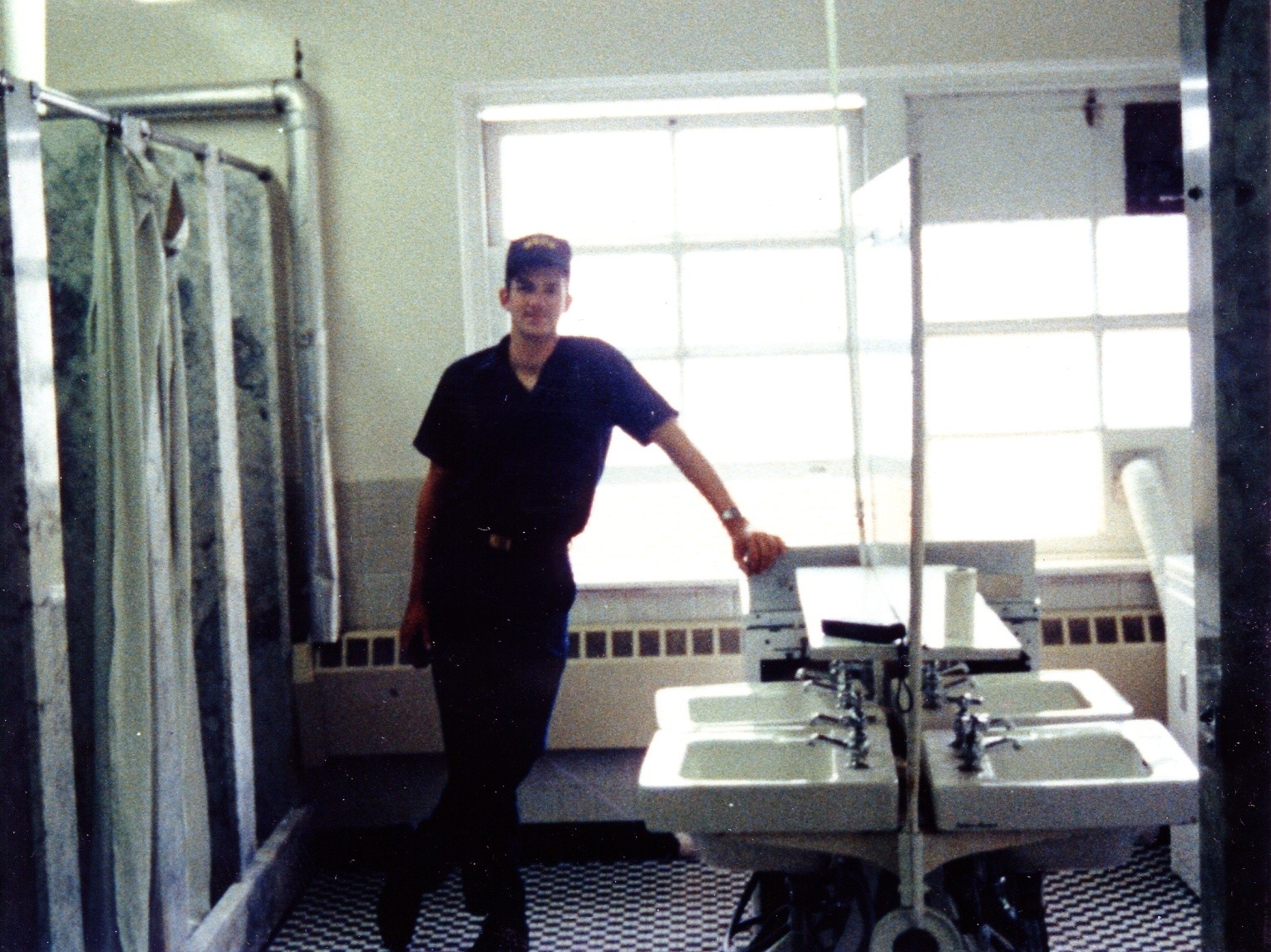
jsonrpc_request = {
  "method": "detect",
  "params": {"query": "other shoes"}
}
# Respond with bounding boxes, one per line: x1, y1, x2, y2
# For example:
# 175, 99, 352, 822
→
379, 821, 420, 952
472, 916, 530, 952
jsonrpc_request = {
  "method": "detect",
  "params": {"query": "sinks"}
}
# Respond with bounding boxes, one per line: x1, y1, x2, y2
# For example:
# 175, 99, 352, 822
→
636, 722, 901, 834
651, 679, 889, 732
890, 666, 1135, 729
920, 717, 1204, 833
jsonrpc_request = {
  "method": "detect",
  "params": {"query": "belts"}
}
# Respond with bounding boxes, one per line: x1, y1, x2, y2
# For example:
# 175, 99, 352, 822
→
465, 527, 528, 557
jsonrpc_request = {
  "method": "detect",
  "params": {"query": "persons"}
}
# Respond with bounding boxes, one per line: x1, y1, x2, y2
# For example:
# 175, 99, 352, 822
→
377, 235, 787, 952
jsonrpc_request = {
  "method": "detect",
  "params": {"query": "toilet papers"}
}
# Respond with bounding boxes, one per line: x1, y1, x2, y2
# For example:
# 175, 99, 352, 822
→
943, 566, 980, 640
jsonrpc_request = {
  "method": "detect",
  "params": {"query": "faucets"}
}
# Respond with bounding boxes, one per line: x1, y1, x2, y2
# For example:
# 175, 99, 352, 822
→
793, 658, 853, 692
920, 663, 970, 684
951, 712, 1024, 775
808, 691, 878, 732
801, 714, 873, 772
802, 679, 856, 711
920, 657, 978, 711
945, 691, 1014, 749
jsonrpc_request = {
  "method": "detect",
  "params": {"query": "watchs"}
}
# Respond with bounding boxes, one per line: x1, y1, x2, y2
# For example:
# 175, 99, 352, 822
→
720, 507, 742, 520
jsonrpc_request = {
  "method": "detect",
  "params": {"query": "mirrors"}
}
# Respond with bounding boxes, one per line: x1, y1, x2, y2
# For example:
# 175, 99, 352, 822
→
915, 79, 1187, 844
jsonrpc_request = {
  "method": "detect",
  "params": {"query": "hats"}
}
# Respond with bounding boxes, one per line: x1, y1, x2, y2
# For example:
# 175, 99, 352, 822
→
506, 234, 572, 274
